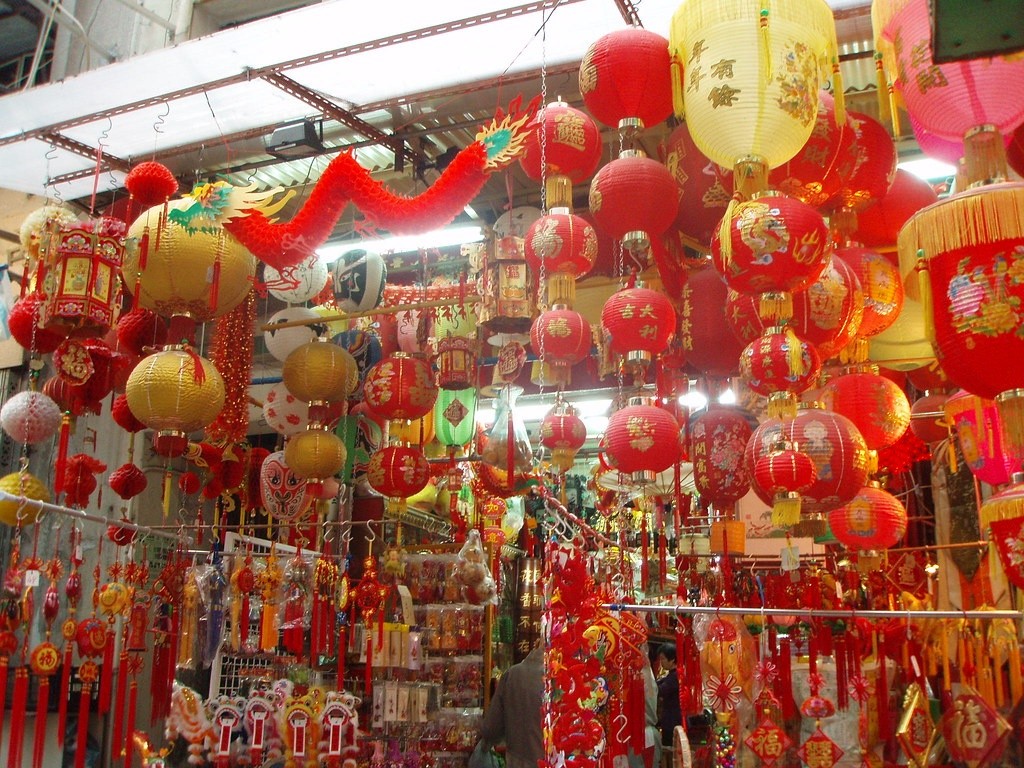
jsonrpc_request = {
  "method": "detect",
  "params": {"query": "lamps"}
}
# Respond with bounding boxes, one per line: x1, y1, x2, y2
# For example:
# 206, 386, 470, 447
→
922, 1, 1024, 64
315, 220, 488, 264
419, 141, 462, 189
265, 120, 326, 161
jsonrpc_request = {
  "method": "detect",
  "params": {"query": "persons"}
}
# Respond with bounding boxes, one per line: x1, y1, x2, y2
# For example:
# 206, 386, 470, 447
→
480, 646, 544, 768
658, 643, 682, 745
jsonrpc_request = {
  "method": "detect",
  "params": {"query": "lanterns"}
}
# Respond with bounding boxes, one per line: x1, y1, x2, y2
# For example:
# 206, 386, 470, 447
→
981, 482, 1024, 590
0, 0, 1024, 568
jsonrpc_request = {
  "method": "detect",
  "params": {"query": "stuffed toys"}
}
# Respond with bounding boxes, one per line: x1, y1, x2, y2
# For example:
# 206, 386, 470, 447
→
165, 680, 362, 768
